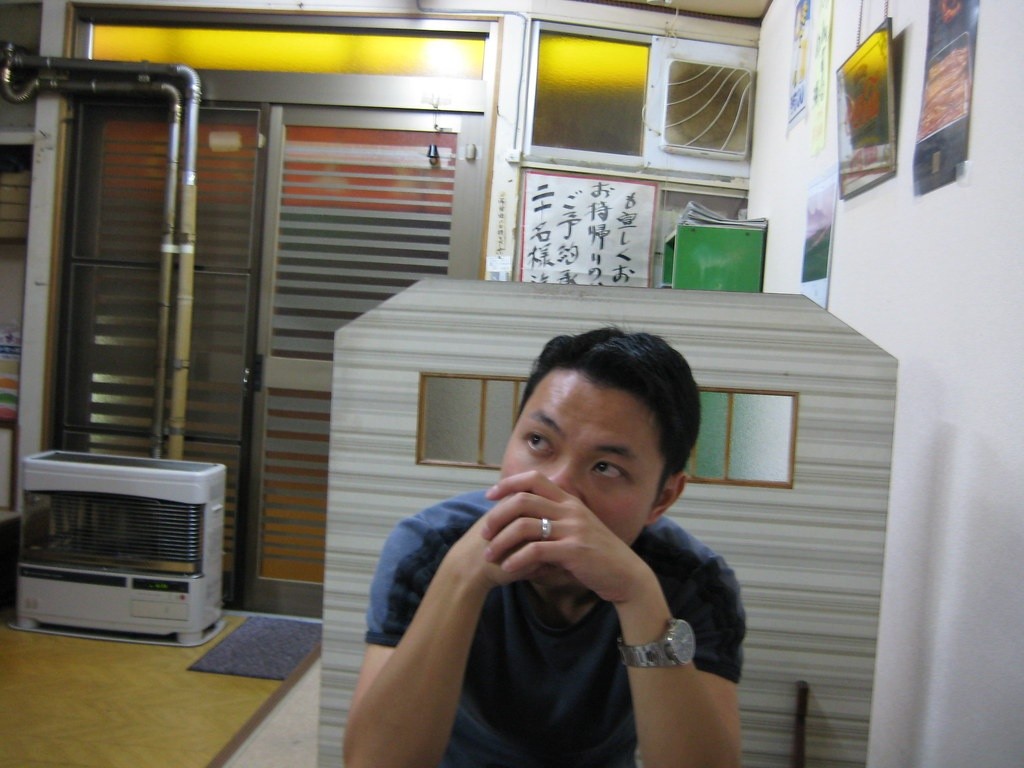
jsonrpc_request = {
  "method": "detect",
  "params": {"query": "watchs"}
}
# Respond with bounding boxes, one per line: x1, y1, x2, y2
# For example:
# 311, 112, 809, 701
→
617, 619, 697, 668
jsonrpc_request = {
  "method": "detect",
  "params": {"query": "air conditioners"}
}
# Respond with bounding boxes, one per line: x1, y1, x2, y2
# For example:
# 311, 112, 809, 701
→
17, 449, 226, 644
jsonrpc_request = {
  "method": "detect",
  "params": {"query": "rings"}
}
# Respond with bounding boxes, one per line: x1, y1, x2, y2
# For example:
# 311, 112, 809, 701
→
541, 518, 552, 540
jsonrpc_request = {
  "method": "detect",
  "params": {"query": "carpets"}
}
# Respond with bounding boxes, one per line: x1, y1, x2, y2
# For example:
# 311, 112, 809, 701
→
186, 615, 321, 680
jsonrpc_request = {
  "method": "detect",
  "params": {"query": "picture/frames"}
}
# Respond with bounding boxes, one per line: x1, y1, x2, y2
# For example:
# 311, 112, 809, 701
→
836, 18, 897, 200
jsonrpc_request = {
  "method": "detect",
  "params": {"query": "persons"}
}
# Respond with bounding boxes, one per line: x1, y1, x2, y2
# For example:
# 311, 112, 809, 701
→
342, 327, 746, 768
